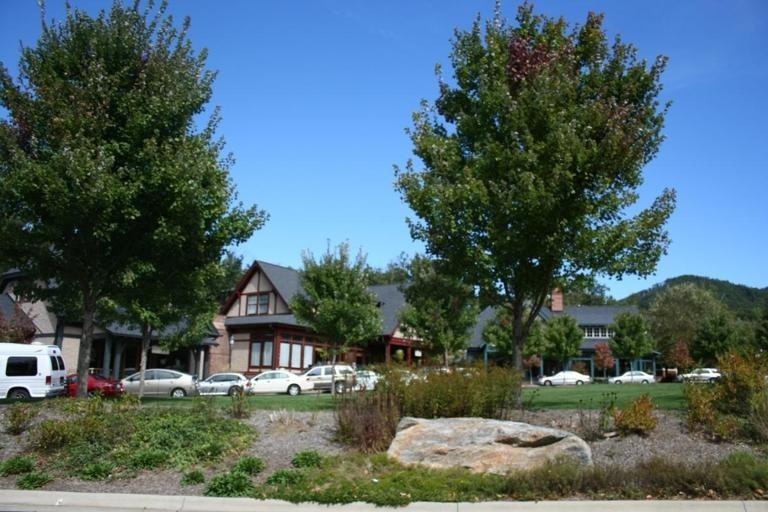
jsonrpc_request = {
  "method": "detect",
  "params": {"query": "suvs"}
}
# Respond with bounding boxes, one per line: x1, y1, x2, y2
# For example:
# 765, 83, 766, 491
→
302, 364, 356, 394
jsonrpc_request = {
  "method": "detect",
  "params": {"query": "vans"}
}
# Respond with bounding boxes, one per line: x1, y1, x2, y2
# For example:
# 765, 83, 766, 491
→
0, 342, 67, 404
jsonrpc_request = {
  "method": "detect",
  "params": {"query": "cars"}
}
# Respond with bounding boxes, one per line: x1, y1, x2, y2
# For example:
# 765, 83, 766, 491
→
64, 372, 123, 399
247, 371, 315, 397
357, 366, 478, 391
677, 368, 720, 385
194, 372, 247, 396
607, 372, 654, 384
539, 371, 591, 387
120, 368, 200, 399
